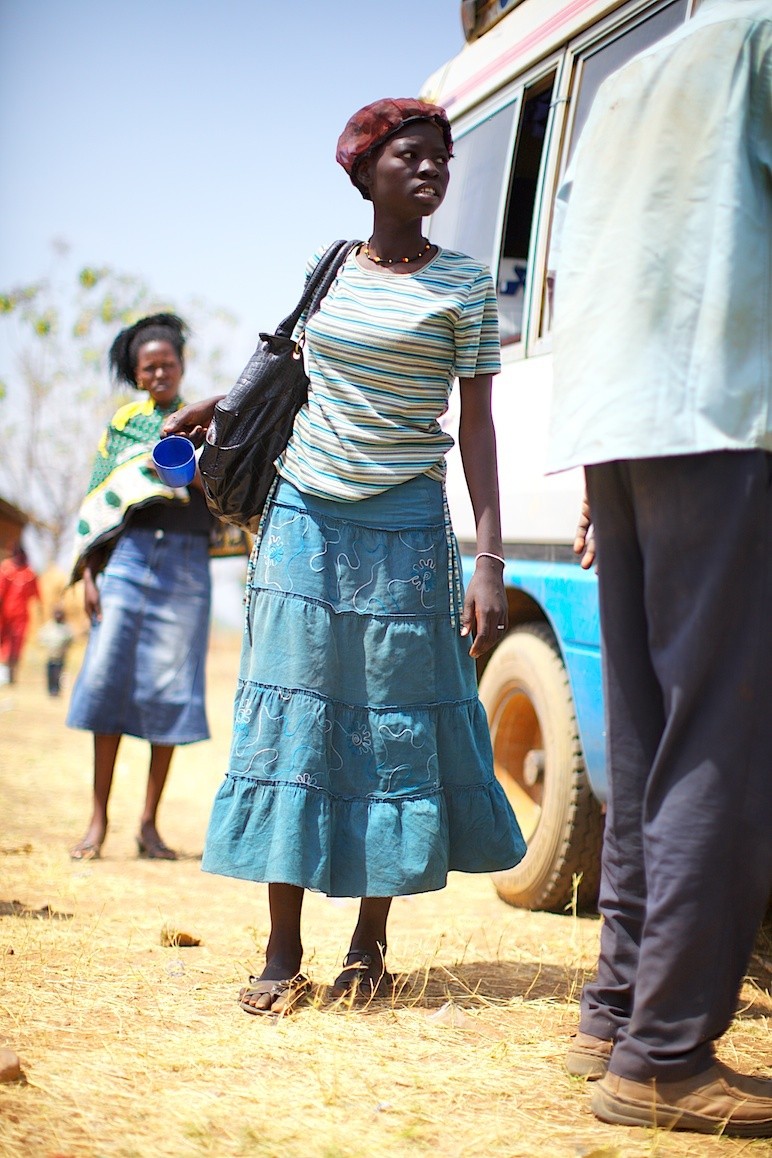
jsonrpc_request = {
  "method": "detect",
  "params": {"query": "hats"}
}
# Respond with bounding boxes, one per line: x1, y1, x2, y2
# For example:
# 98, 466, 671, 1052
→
335, 97, 453, 200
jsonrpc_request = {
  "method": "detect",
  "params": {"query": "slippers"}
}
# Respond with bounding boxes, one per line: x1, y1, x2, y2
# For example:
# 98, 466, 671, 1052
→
240, 972, 310, 1019
330, 949, 393, 999
137, 837, 177, 860
71, 840, 102, 860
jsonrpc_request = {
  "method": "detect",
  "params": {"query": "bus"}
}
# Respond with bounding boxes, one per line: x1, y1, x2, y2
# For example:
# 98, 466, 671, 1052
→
413, 0, 764, 913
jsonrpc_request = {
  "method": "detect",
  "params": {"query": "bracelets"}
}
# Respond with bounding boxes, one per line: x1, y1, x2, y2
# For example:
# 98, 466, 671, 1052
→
475, 552, 506, 569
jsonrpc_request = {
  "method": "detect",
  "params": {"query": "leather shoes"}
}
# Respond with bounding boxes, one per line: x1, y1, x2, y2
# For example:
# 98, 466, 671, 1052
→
567, 1032, 617, 1079
594, 1053, 771, 1136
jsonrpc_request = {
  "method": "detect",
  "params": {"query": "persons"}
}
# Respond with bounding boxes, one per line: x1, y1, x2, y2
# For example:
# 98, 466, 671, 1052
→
160, 98, 526, 1013
67, 312, 212, 861
0, 547, 71, 696
543, 0, 772, 1132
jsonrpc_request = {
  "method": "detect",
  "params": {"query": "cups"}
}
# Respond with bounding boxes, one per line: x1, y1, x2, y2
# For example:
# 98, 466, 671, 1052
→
151, 432, 196, 489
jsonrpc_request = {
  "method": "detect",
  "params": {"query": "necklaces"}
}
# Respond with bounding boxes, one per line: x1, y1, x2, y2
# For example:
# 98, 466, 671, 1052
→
365, 236, 431, 264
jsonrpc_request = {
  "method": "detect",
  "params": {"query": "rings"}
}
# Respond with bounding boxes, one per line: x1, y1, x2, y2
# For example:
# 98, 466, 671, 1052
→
498, 626, 504, 629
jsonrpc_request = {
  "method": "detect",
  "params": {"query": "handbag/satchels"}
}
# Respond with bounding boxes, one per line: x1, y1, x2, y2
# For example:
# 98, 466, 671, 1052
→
198, 239, 365, 535
